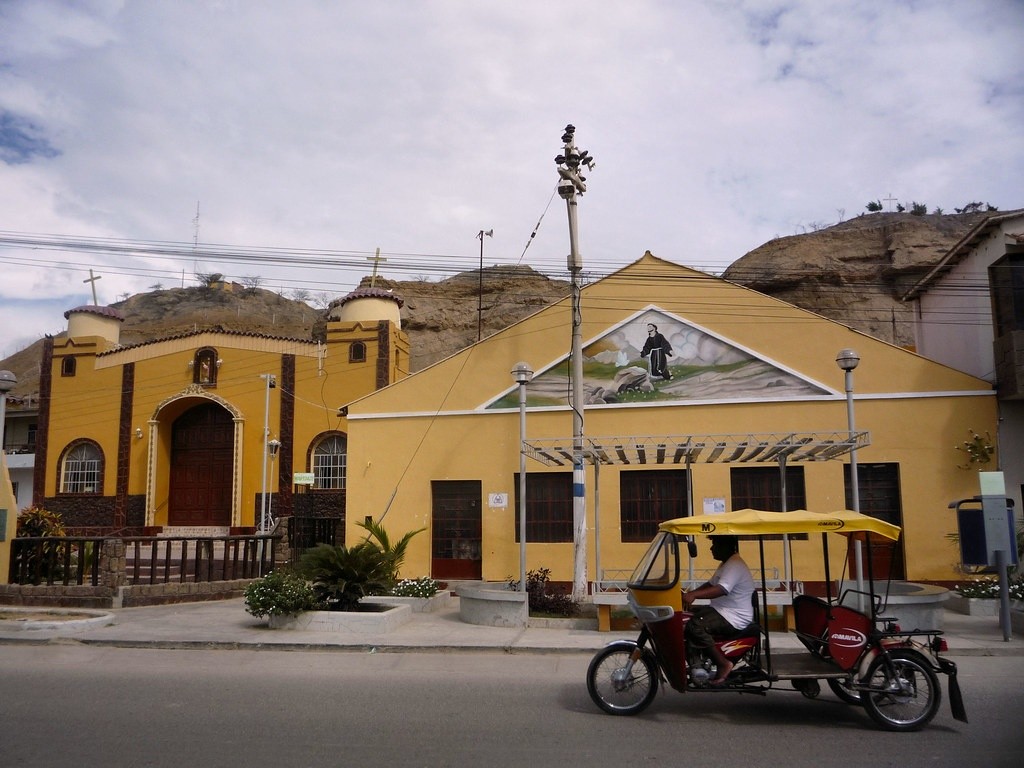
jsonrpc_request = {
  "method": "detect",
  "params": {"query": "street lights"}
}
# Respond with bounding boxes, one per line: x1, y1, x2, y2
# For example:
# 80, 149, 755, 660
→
510, 362, 534, 591
836, 348, 866, 613
267, 439, 282, 533
0, 370, 18, 468
559, 178, 589, 607
258, 374, 277, 576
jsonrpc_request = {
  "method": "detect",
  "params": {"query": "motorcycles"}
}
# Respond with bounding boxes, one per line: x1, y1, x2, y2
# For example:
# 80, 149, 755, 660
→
587, 509, 969, 732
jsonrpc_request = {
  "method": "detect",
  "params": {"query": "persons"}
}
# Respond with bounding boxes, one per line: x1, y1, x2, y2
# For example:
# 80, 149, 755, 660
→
683, 536, 756, 686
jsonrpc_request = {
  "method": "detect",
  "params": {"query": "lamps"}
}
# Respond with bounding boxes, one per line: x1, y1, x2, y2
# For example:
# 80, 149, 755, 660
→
216, 359, 222, 367
136, 428, 143, 439
189, 361, 193, 368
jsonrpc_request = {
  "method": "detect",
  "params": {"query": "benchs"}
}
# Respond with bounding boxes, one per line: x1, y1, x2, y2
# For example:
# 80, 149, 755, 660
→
591, 570, 804, 633
790, 594, 871, 671
